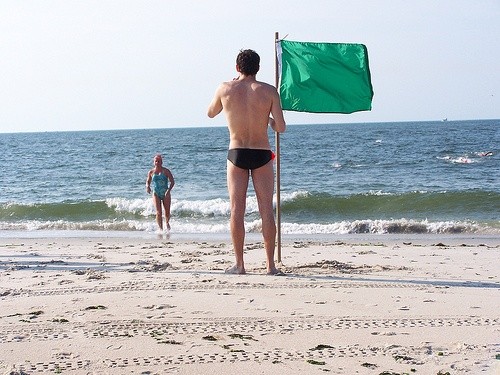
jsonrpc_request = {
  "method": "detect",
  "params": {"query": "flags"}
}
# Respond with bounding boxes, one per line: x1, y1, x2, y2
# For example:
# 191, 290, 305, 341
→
275, 38, 374, 115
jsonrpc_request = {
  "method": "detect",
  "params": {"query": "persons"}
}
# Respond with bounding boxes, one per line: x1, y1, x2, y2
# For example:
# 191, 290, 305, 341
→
146, 155, 174, 230
207, 49, 286, 275
443, 151, 492, 164
